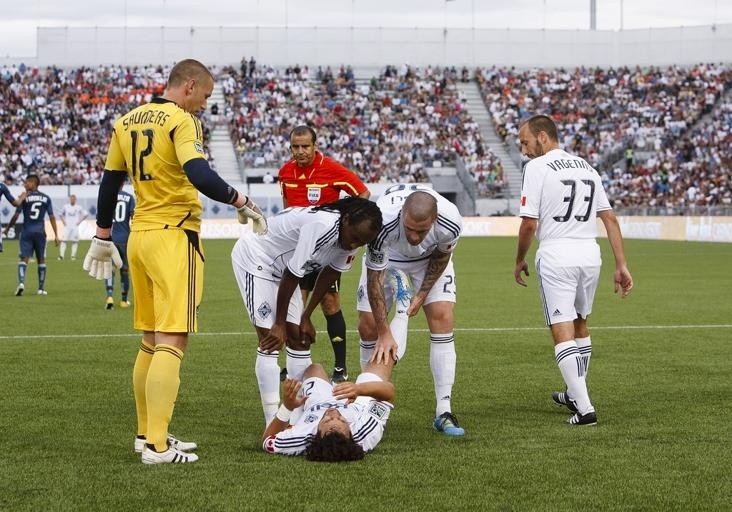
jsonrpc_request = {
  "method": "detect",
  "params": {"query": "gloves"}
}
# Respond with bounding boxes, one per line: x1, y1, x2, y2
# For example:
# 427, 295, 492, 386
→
83, 236, 123, 280
237, 196, 268, 236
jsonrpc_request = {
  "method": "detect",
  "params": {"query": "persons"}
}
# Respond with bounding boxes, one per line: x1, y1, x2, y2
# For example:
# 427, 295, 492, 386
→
209, 57, 507, 199
82, 60, 269, 465
76, 183, 136, 311
514, 115, 633, 427
0, 182, 28, 252
0, 60, 178, 184
277, 126, 370, 387
231, 196, 383, 430
2, 175, 61, 297
58, 195, 91, 262
355, 183, 466, 436
260, 267, 416, 461
197, 103, 218, 161
503, 60, 731, 216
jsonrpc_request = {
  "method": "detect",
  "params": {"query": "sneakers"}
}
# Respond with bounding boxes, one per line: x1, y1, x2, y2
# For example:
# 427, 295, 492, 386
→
434, 412, 464, 435
133, 434, 197, 453
36, 290, 46, 295
567, 410, 598, 425
553, 389, 578, 409
16, 284, 24, 296
70, 256, 76, 260
120, 301, 130, 308
106, 298, 113, 309
332, 368, 348, 385
143, 443, 198, 465
58, 257, 64, 260
